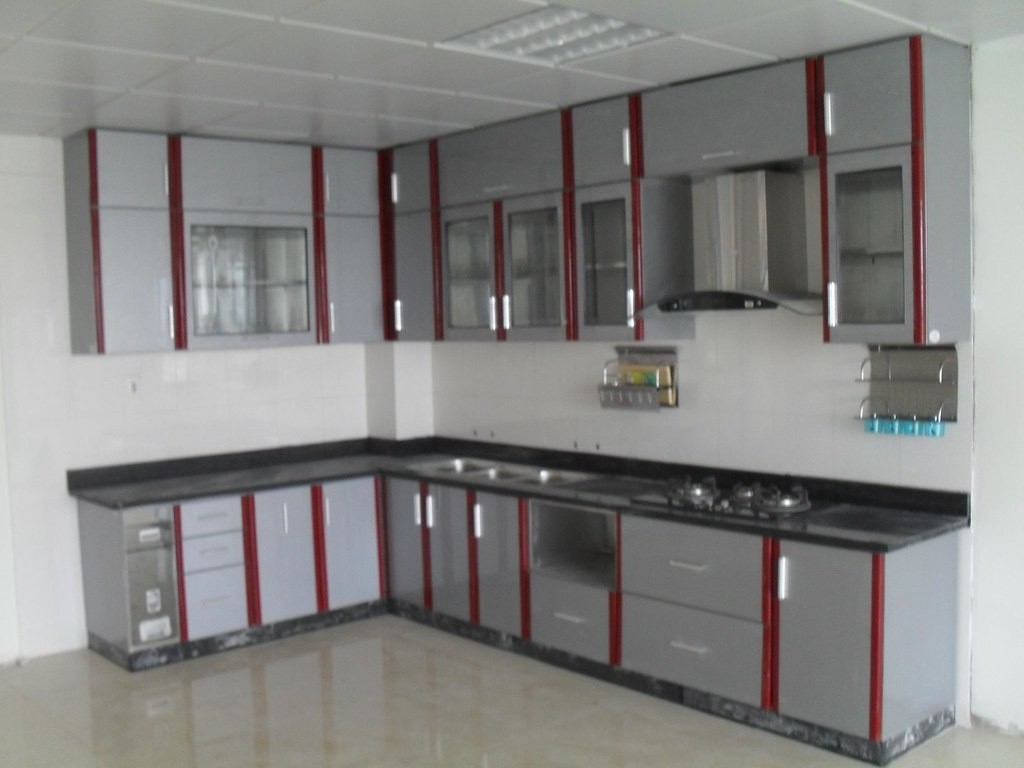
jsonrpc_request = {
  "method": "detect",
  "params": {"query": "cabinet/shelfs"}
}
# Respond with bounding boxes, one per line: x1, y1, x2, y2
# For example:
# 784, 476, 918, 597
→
566, 94, 643, 342
633, 56, 819, 179
384, 475, 475, 626
474, 490, 527, 639
173, 495, 256, 643
527, 497, 618, 667
813, 36, 927, 346
247, 477, 384, 626
434, 110, 573, 343
616, 514, 773, 709
63, 127, 392, 356
385, 139, 442, 342
770, 535, 884, 741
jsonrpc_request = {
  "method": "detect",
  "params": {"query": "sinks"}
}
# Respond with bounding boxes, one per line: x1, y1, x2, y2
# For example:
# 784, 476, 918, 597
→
514, 469, 600, 490
458, 462, 537, 487
402, 458, 496, 480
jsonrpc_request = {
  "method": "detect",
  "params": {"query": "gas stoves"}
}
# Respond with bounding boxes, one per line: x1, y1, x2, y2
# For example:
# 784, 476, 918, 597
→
630, 475, 812, 520
